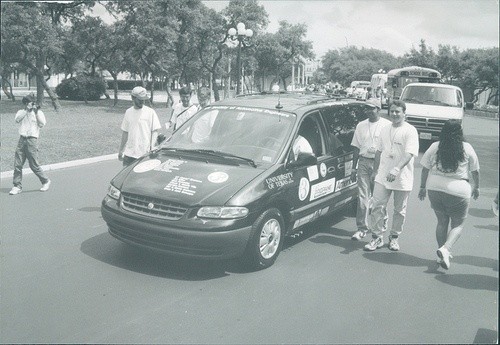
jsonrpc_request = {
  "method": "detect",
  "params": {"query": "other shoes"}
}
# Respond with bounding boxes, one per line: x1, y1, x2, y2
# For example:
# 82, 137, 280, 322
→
352, 230, 367, 239
9, 187, 22, 195
491, 201, 499, 216
40, 181, 50, 191
437, 245, 452, 270
382, 217, 388, 231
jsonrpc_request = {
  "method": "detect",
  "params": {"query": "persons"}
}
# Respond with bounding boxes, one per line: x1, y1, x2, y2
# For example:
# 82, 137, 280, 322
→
304, 82, 383, 100
118, 86, 162, 169
167, 86, 197, 131
9, 95, 52, 195
349, 98, 392, 241
417, 122, 480, 269
364, 100, 419, 251
263, 122, 314, 163
287, 82, 296, 91
186, 86, 219, 144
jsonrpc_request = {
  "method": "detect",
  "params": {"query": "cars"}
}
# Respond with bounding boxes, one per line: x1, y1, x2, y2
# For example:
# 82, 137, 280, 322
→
100, 92, 369, 268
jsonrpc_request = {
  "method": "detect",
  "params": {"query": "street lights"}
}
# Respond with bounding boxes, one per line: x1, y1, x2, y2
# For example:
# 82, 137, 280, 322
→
228, 22, 254, 94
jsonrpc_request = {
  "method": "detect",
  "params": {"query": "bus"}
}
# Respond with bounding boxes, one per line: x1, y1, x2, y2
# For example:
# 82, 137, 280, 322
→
345, 73, 388, 109
385, 65, 442, 116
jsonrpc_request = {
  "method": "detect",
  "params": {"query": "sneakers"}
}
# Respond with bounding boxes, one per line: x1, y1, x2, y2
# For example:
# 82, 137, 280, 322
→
387, 234, 401, 250
365, 237, 384, 250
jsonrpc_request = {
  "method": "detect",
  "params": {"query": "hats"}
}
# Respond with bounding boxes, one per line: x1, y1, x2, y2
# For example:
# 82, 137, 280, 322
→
132, 85, 150, 100
24, 92, 36, 102
365, 98, 382, 109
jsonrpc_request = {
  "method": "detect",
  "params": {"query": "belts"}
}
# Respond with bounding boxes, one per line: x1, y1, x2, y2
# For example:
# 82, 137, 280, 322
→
21, 136, 37, 139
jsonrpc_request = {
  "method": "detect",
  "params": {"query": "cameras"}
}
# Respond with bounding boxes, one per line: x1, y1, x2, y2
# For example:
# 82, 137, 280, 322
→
32, 102, 40, 109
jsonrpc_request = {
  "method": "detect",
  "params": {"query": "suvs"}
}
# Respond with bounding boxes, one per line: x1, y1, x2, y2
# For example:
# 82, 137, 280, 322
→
392, 82, 475, 153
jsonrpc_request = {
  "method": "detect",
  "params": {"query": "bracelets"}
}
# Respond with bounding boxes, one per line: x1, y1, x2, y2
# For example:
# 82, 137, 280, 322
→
420, 186, 425, 188
351, 169, 357, 172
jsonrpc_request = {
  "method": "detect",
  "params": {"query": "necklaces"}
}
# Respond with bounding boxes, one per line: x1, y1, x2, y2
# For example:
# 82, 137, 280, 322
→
389, 126, 399, 158
